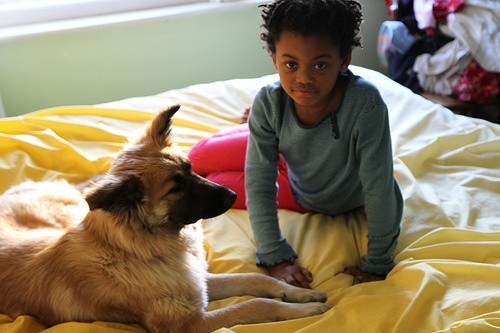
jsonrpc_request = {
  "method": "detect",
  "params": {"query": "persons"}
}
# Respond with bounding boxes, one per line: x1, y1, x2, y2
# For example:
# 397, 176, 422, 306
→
186, 0, 403, 290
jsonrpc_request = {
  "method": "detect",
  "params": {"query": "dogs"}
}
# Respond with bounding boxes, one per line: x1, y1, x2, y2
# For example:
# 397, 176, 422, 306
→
0, 105, 334, 333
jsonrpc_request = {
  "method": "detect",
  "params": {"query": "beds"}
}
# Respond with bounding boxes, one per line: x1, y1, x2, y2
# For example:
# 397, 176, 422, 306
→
0, 63, 500, 333
